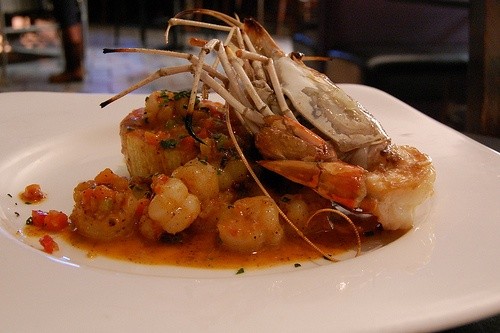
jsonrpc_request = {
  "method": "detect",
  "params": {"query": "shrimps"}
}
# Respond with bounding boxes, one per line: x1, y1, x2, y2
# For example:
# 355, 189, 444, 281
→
100, 9, 437, 262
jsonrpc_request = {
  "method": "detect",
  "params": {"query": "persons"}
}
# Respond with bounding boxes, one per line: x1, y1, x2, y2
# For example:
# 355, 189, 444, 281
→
47, 0, 85, 83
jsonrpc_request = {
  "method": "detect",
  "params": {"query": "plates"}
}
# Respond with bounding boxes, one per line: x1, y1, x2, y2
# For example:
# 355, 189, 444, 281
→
0, 84, 500, 332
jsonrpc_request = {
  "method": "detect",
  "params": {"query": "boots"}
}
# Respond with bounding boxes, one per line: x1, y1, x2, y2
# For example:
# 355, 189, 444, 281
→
50, 42, 83, 82
